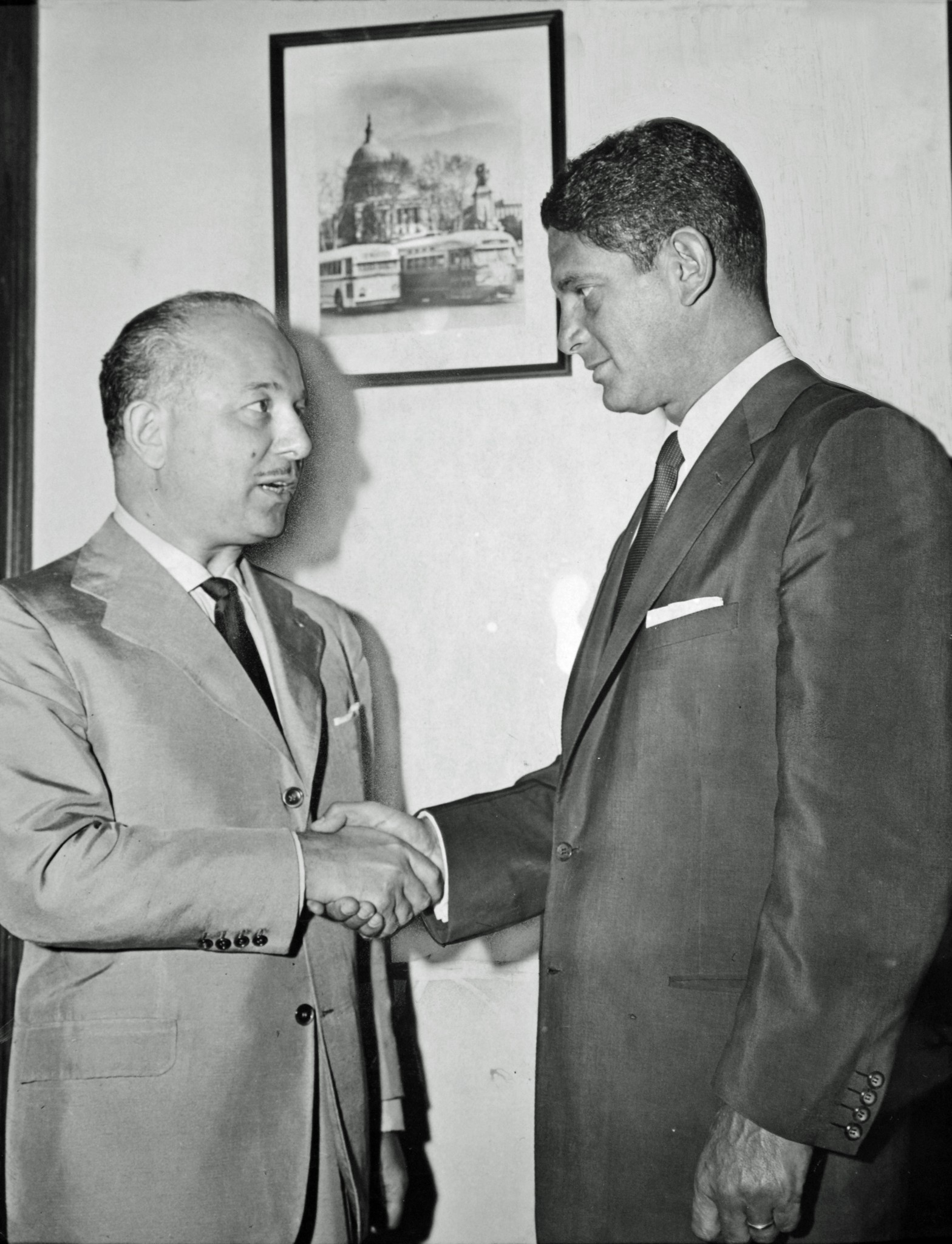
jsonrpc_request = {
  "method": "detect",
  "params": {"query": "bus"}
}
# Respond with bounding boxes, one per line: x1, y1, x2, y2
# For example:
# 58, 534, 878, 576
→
401, 227, 522, 308
319, 241, 402, 316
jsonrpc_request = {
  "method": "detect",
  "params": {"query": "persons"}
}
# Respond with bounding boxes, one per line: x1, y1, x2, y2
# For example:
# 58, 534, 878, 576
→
0, 291, 443, 1242
304, 117, 952, 1244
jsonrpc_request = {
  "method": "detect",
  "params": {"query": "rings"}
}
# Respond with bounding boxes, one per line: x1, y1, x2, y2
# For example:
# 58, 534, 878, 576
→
747, 1219, 774, 1230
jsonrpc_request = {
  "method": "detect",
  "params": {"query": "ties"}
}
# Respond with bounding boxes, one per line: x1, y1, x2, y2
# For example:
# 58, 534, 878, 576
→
610, 432, 682, 633
199, 576, 282, 735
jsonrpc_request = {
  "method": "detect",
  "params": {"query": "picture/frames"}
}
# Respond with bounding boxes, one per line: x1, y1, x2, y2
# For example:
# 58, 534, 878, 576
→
270, 7, 573, 394
